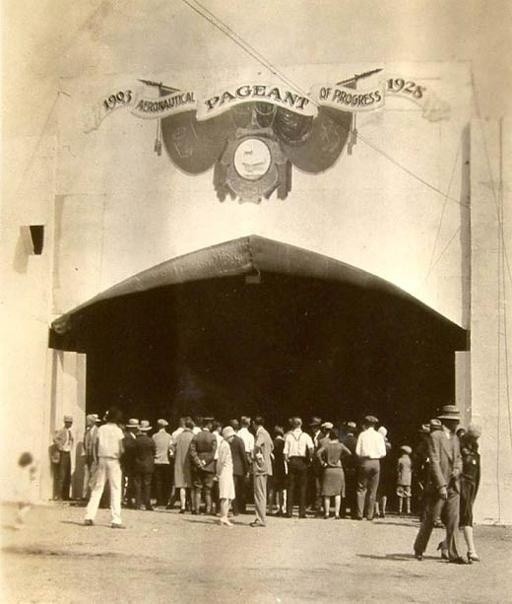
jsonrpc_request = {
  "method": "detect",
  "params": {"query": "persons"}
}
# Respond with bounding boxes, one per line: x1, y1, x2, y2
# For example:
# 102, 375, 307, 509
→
247, 420, 274, 528
84, 409, 127, 529
214, 425, 237, 527
414, 405, 471, 564
437, 426, 482, 561
53, 415, 392, 519
398, 410, 467, 528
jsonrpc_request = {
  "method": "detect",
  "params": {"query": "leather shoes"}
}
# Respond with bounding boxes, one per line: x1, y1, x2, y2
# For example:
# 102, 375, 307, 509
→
250, 522, 266, 527
84, 518, 94, 526
414, 547, 424, 562
111, 522, 126, 529
449, 557, 473, 564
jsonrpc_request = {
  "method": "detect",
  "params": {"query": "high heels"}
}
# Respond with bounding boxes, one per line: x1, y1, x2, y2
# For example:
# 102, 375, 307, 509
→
436, 541, 450, 560
467, 551, 481, 563
373, 512, 385, 519
219, 517, 234, 526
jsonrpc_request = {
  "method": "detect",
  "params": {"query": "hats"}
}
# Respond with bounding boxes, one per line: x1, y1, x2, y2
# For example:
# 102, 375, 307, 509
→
308, 416, 324, 426
63, 410, 168, 431
320, 421, 334, 429
400, 444, 412, 454
202, 415, 216, 422
362, 415, 379, 425
435, 404, 465, 420
222, 426, 236, 441
